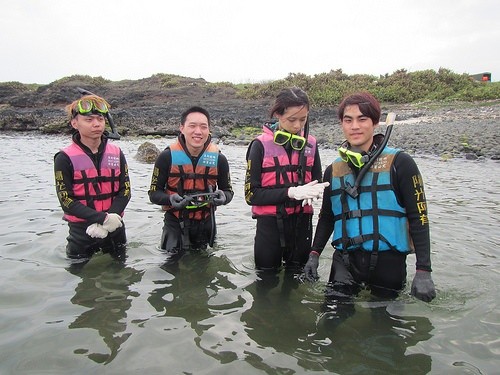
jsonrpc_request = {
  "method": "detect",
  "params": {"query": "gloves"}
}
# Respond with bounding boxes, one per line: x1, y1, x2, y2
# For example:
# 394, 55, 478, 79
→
102, 213, 123, 233
86, 223, 108, 239
289, 179, 330, 207
169, 192, 189, 209
410, 270, 436, 303
304, 253, 319, 282
209, 185, 226, 206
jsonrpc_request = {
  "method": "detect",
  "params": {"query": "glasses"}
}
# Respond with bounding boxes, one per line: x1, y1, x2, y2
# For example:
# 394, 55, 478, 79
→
337, 146, 369, 168
273, 130, 306, 151
76, 98, 108, 116
185, 193, 210, 209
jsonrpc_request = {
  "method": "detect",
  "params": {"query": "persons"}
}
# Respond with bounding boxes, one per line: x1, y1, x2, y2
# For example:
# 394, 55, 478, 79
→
245, 87, 329, 269
53, 96, 131, 258
304, 93, 436, 302
148, 106, 234, 253
